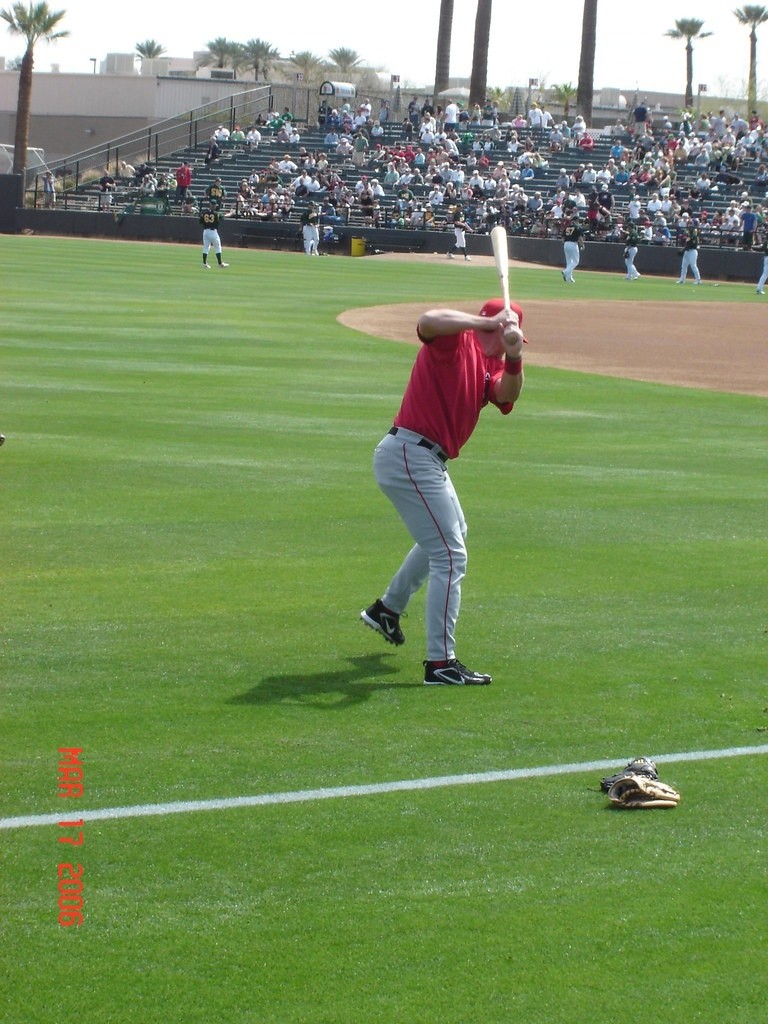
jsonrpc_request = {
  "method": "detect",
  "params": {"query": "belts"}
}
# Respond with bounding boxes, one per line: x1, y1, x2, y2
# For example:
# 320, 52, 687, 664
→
389, 426, 449, 464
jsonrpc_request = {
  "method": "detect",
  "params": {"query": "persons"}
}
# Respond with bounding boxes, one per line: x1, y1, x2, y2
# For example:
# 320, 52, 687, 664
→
754, 238, 768, 295
42, 171, 57, 210
624, 222, 640, 280
300, 200, 320, 256
446, 203, 472, 261
199, 198, 229, 269
120, 162, 136, 177
134, 99, 768, 250
360, 298, 528, 689
561, 216, 585, 283
100, 169, 115, 211
676, 227, 701, 285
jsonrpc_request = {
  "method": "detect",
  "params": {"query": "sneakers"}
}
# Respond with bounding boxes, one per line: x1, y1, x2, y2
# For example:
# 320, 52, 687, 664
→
360, 599, 406, 646
422, 657, 492, 685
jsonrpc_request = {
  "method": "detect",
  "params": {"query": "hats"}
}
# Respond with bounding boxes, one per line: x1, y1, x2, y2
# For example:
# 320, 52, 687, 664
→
38, 90, 767, 237
479, 298, 528, 344
210, 198, 217, 204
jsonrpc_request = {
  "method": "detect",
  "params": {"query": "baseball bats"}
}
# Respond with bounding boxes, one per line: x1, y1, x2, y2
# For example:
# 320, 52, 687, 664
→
490, 224, 518, 345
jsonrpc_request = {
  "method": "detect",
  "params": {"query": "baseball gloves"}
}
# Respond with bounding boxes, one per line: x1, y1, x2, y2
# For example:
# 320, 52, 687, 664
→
676, 251, 684, 257
624, 251, 629, 258
587, 754, 682, 809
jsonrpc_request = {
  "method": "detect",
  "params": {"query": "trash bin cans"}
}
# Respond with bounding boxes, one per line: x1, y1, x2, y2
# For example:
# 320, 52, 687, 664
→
351, 238, 366, 256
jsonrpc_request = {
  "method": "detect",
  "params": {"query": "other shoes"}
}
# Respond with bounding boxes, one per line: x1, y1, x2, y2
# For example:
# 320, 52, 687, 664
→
203, 263, 211, 268
447, 252, 455, 258
218, 263, 229, 267
693, 280, 701, 285
675, 280, 684, 284
635, 274, 641, 280
757, 290, 765, 295
561, 272, 575, 282
465, 256, 471, 261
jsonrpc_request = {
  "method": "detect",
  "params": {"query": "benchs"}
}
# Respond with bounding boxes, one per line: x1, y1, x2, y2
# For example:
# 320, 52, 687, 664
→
56, 119, 768, 250
